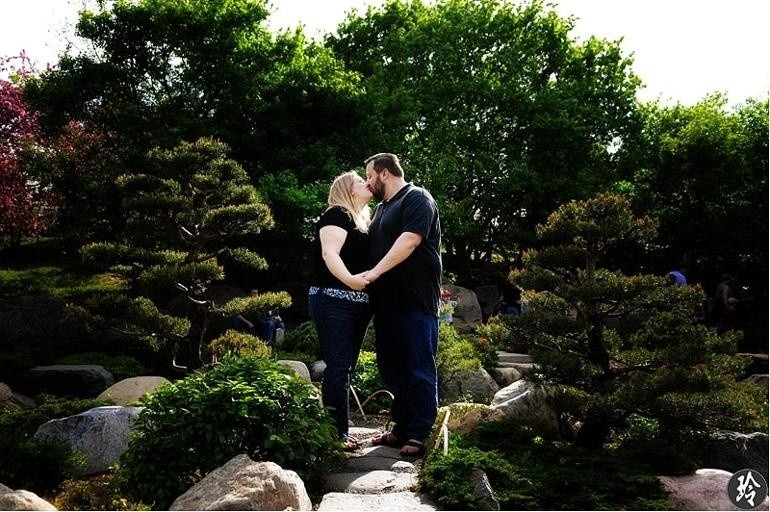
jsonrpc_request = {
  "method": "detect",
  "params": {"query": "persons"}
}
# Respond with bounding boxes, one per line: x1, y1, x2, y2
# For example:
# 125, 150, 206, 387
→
507, 297, 525, 318
439, 290, 461, 325
238, 288, 285, 346
664, 270, 687, 290
714, 275, 734, 329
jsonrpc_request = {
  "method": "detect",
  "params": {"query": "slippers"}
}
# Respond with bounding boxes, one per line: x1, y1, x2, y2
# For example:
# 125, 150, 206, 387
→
340, 435, 358, 450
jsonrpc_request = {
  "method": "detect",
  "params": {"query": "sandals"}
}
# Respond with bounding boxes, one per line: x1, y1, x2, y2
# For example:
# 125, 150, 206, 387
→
372, 432, 426, 457
